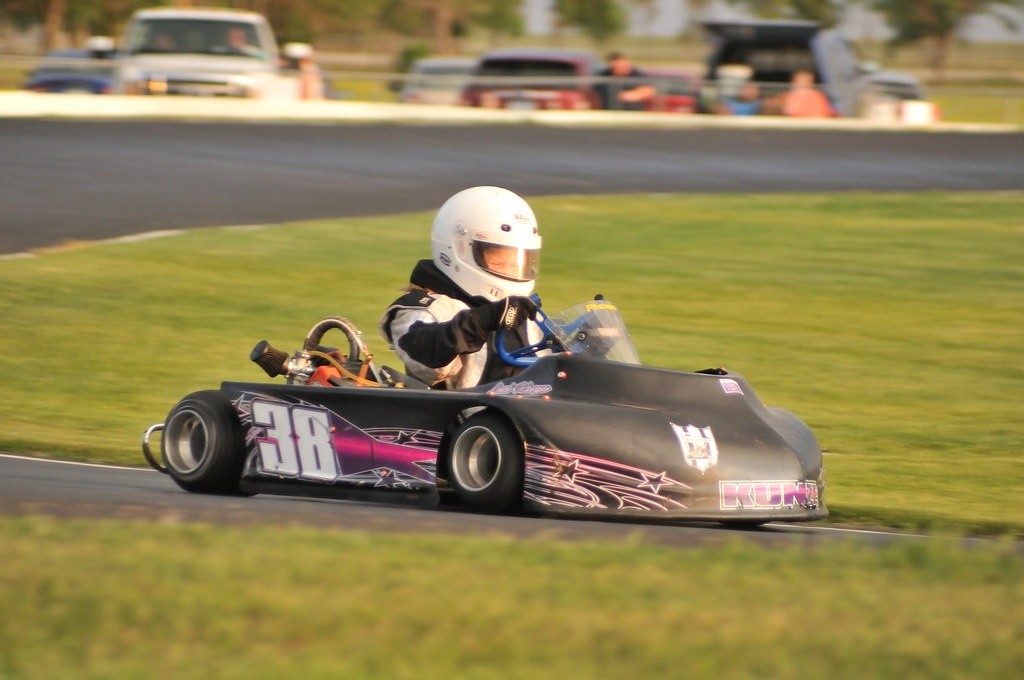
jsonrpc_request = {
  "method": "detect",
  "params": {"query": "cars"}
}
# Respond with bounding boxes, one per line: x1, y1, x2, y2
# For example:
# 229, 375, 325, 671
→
402, 45, 607, 113
26, 50, 114, 93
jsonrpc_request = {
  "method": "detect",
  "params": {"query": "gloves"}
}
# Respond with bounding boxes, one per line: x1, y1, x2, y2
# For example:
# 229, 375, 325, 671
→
473, 295, 540, 333
594, 293, 604, 301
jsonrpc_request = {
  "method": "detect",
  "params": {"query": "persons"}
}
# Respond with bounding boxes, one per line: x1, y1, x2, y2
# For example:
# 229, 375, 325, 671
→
380, 187, 618, 391
712, 81, 778, 116
147, 31, 178, 52
590, 52, 655, 111
782, 69, 833, 117
209, 28, 263, 57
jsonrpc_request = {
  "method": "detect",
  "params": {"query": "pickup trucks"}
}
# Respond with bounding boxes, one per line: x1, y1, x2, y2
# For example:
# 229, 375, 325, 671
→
113, 5, 324, 99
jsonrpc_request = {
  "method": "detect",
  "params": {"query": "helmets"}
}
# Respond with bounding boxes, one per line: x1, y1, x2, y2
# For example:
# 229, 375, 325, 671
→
431, 185, 542, 302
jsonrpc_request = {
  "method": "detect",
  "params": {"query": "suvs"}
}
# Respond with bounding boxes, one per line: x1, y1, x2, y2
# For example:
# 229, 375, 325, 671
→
699, 18, 923, 114
